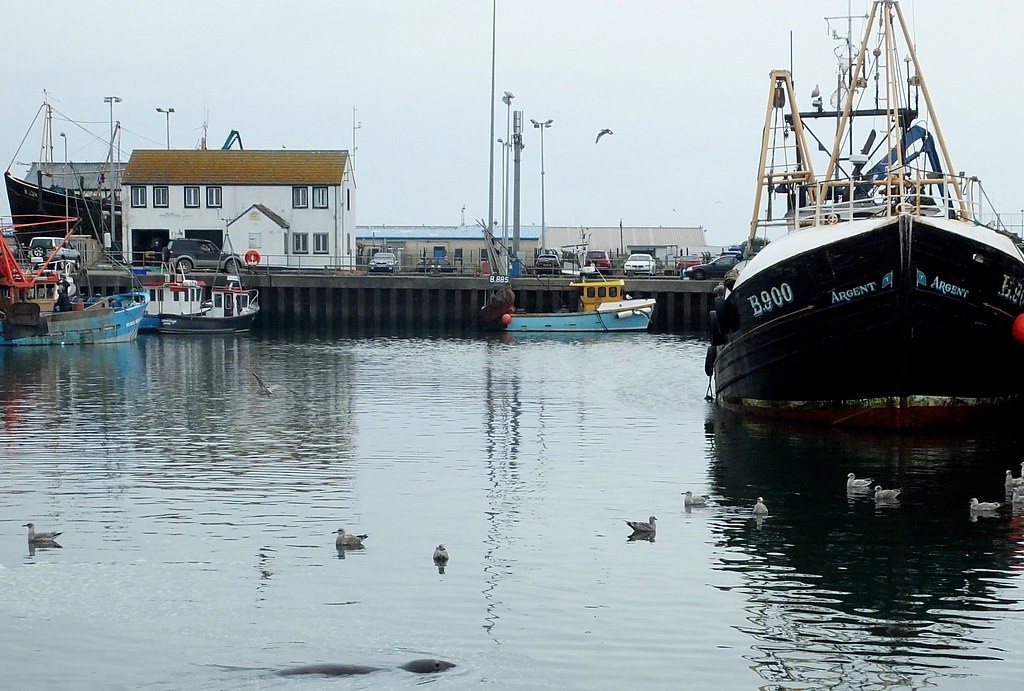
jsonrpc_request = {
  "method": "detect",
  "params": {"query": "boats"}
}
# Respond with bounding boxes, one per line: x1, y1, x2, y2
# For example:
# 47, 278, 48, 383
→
472, 219, 656, 331
702, 2, 1024, 428
0, 89, 261, 345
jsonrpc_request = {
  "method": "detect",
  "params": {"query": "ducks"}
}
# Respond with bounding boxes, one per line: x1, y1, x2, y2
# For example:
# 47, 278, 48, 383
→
875, 485, 901, 499
847, 471, 874, 489
625, 517, 658, 533
969, 497, 1001, 511
1005, 462, 1024, 502
752, 496, 768, 515
22, 523, 65, 541
331, 528, 369, 546
682, 491, 713, 507
433, 544, 450, 561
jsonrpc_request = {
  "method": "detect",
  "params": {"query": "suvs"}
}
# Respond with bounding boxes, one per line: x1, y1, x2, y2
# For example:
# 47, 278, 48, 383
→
162, 238, 245, 274
27, 237, 81, 270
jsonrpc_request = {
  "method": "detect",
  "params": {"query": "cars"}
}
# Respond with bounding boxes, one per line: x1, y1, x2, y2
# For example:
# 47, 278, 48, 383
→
677, 247, 743, 279
586, 249, 612, 272
535, 254, 562, 275
367, 252, 398, 273
623, 254, 657, 275
416, 257, 457, 274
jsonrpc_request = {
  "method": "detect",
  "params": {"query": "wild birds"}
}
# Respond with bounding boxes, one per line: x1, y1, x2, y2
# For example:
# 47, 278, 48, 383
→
244, 367, 273, 398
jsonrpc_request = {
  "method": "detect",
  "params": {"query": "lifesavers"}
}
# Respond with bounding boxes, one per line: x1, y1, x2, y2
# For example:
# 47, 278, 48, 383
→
704, 347, 717, 376
715, 296, 731, 335
709, 310, 723, 346
245, 250, 260, 265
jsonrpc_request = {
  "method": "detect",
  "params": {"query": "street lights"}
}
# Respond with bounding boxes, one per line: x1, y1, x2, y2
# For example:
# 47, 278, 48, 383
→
103, 96, 124, 241
496, 91, 514, 255
59, 133, 69, 236
530, 119, 553, 253
156, 107, 174, 150
